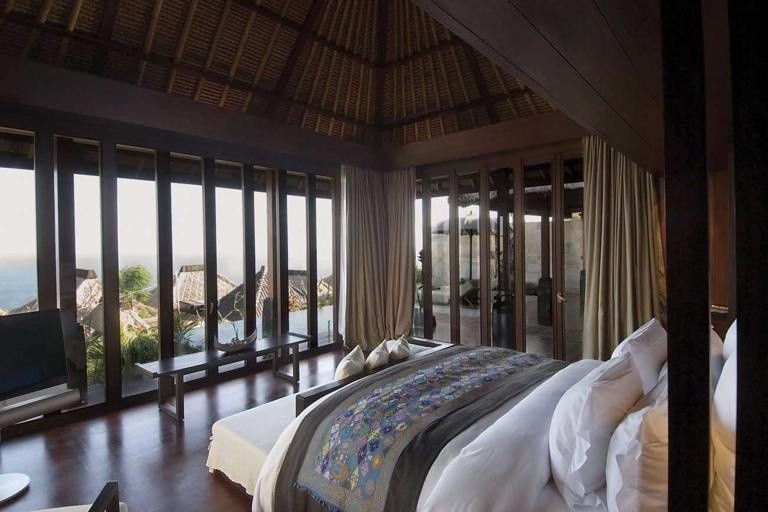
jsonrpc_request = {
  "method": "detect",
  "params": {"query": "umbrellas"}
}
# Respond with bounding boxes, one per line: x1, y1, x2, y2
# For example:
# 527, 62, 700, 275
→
432, 210, 513, 280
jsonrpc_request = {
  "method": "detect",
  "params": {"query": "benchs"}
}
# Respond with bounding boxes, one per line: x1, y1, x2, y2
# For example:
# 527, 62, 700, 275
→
207, 335, 454, 495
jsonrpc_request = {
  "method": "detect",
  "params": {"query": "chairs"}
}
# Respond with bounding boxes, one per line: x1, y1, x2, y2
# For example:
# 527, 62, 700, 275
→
416, 275, 500, 309
0, 478, 130, 512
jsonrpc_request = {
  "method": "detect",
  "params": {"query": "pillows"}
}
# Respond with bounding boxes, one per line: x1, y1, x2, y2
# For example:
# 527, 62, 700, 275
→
548, 353, 643, 511
611, 316, 669, 394
334, 345, 365, 380
658, 321, 725, 387
605, 367, 716, 511
364, 338, 390, 370
390, 335, 411, 361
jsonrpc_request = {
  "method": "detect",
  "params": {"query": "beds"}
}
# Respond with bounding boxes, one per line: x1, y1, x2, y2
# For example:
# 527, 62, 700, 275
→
250, 317, 736, 512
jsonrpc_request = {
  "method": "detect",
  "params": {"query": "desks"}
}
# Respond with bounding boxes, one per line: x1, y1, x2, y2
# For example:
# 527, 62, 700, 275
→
134, 332, 312, 420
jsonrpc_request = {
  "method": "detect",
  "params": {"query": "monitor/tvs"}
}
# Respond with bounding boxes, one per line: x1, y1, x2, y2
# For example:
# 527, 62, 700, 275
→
0, 308, 68, 410
